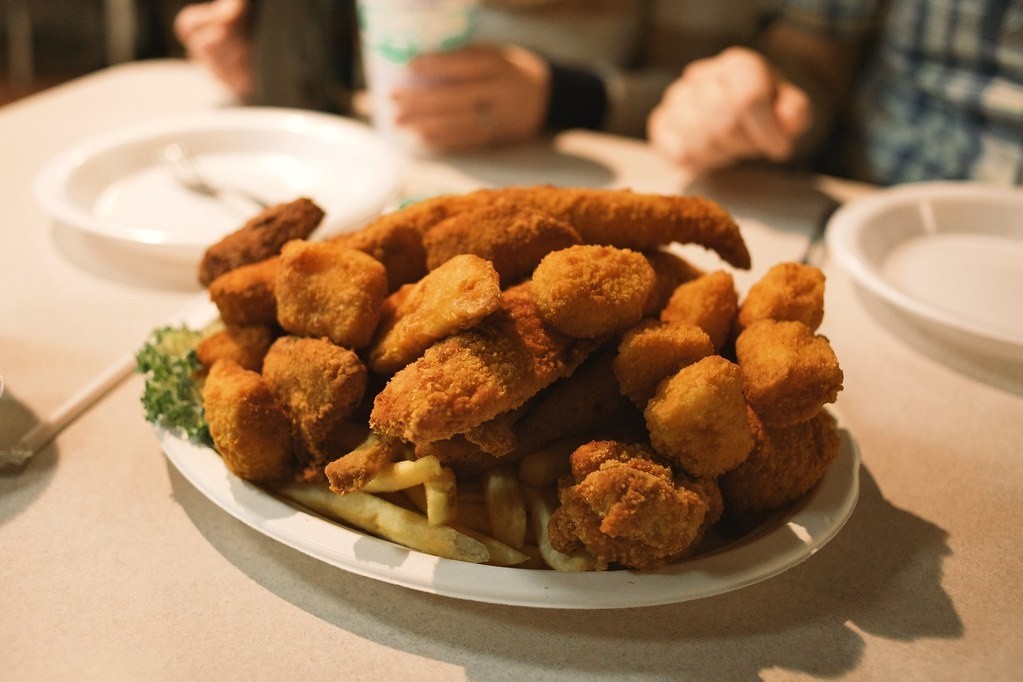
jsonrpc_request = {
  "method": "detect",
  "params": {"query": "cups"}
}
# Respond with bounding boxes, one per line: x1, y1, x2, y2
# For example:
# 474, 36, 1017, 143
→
356, 1, 483, 144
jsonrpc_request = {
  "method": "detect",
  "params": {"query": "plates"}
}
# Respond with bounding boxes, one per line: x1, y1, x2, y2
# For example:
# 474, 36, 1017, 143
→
152, 315, 862, 610
824, 182, 1023, 366
28, 109, 403, 260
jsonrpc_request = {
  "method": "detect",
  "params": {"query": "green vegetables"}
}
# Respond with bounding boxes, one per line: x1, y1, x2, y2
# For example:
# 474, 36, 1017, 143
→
134, 323, 212, 448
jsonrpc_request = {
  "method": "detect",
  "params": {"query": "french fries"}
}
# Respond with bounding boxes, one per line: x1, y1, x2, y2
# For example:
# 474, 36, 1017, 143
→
279, 447, 594, 571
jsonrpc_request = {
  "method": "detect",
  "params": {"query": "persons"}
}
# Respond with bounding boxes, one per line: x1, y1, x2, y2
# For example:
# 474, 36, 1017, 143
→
172, 0, 1023, 196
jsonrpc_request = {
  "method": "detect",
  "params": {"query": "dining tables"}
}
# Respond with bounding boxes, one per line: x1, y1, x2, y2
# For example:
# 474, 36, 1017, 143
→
0, 60, 1023, 682
